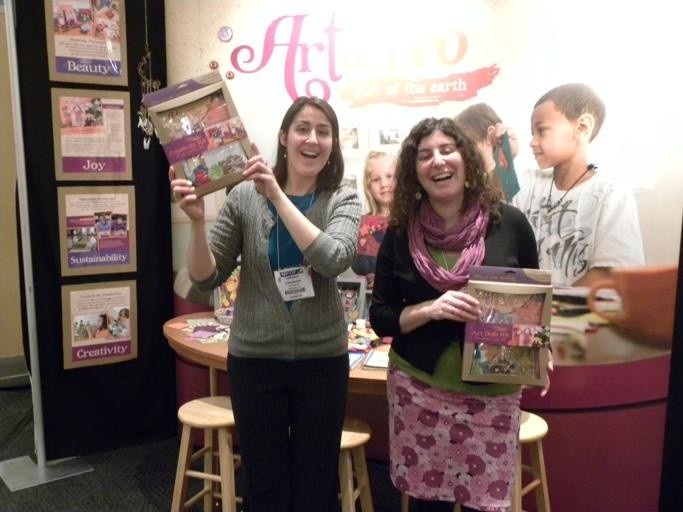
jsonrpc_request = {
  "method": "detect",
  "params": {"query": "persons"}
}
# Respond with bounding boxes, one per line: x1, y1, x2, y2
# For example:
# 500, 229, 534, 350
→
165, 97, 362, 512
354, 150, 401, 275
368, 118, 554, 509
454, 102, 502, 171
511, 82, 647, 287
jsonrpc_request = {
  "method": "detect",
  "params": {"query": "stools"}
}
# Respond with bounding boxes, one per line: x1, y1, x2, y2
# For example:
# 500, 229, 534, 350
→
338, 417, 374, 512
171, 396, 243, 512
454, 410, 551, 512
